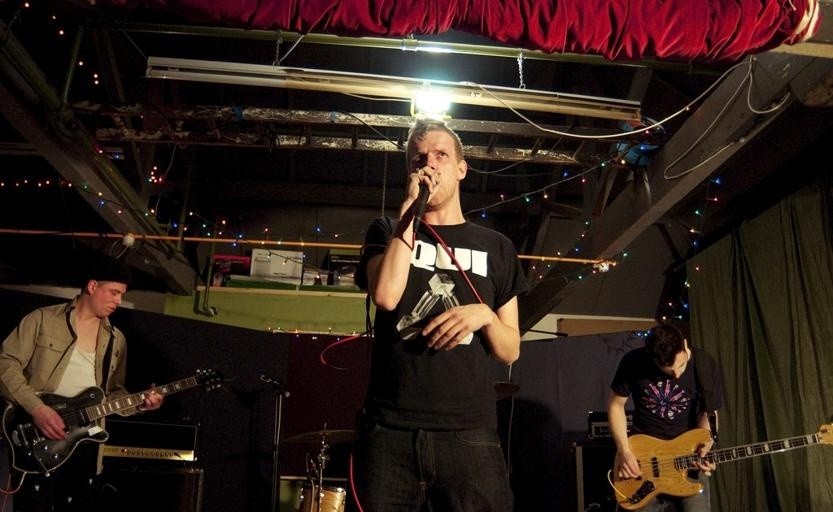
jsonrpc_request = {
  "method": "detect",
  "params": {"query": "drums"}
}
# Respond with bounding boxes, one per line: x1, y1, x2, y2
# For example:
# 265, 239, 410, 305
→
299, 484, 346, 511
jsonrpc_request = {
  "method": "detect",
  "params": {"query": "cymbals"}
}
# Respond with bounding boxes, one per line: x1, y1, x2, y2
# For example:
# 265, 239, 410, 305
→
284, 430, 353, 444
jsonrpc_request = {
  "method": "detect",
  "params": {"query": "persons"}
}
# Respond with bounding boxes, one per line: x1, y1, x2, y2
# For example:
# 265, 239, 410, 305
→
606, 325, 725, 512
353, 119, 531, 511
0, 256, 166, 511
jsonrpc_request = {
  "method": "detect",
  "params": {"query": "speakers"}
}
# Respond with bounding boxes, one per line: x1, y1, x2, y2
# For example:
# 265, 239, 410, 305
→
575, 438, 625, 512
95, 465, 205, 511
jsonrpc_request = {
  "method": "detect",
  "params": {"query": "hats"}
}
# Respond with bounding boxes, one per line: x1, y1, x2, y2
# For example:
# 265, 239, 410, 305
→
84, 252, 131, 287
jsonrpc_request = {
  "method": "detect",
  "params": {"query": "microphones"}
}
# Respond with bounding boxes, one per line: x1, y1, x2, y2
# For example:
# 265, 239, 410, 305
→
256, 373, 291, 398
413, 183, 430, 232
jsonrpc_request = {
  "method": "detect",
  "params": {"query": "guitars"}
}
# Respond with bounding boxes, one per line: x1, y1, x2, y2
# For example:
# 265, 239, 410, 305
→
3, 367, 223, 473
614, 422, 833, 509
